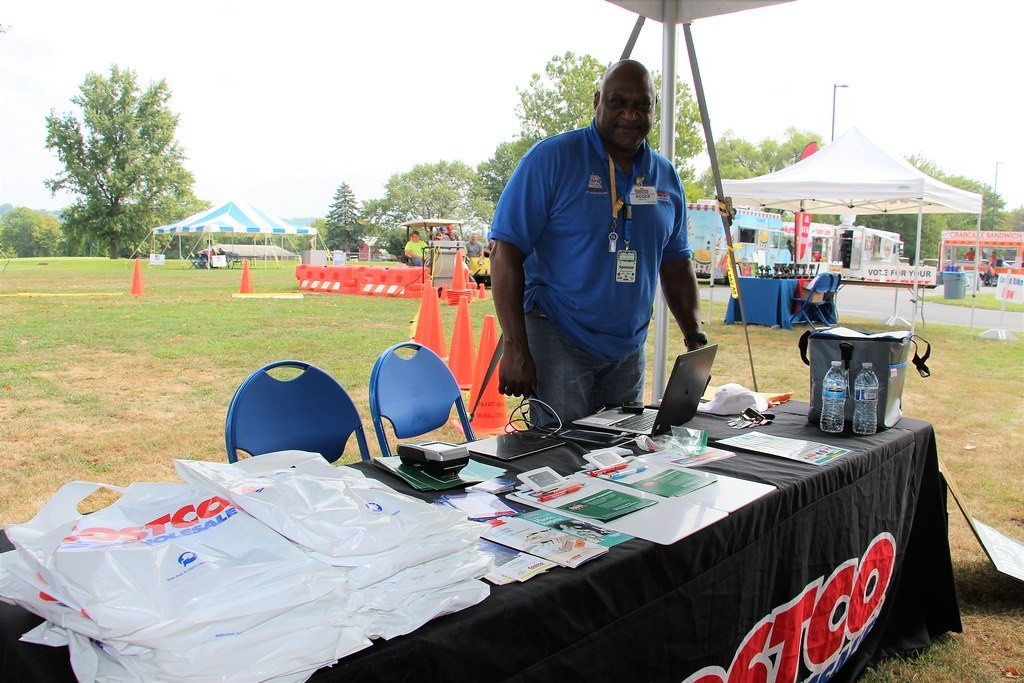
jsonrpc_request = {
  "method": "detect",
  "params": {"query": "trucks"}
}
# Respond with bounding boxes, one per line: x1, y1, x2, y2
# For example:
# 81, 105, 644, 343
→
684, 199, 791, 284
856, 225, 905, 267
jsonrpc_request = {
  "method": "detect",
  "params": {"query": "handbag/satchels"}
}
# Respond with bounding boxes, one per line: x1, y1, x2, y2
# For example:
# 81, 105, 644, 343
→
0, 450, 495, 683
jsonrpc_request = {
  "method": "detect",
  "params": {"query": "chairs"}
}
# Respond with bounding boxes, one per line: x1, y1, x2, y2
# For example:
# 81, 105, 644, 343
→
369, 342, 477, 457
790, 273, 840, 329
225, 360, 371, 466
399, 247, 416, 265
187, 253, 243, 269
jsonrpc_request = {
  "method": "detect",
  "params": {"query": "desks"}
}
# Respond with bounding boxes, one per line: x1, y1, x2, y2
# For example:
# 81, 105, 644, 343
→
723, 276, 837, 331
0, 396, 962, 683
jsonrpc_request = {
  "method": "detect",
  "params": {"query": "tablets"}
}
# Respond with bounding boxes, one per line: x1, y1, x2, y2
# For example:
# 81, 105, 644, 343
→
557, 429, 624, 446
461, 433, 565, 460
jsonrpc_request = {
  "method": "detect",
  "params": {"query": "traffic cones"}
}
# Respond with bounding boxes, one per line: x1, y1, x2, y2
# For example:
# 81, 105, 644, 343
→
479, 283, 488, 299
129, 257, 147, 295
239, 259, 255, 293
438, 284, 449, 300
407, 282, 449, 360
448, 314, 522, 436
446, 251, 477, 306
446, 297, 478, 390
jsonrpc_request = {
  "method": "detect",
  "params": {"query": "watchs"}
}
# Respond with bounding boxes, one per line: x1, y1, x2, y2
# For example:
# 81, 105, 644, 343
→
684, 331, 707, 345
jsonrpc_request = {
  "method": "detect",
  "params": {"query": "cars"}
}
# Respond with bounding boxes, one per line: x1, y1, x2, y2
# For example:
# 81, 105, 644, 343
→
923, 258, 1017, 287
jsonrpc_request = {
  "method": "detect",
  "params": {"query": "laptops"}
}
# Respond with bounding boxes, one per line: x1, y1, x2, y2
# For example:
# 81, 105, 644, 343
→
572, 344, 719, 436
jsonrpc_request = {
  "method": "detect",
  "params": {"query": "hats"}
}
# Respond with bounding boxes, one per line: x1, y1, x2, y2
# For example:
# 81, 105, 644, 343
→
440, 225, 459, 234
409, 231, 419, 236
813, 254, 820, 261
704, 383, 768, 415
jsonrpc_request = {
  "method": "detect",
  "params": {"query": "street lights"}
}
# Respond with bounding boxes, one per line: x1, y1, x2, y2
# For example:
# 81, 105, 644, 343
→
831, 84, 850, 141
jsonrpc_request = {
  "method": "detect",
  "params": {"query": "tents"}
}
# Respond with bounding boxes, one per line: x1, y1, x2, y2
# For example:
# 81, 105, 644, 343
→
713, 124, 982, 331
152, 196, 319, 270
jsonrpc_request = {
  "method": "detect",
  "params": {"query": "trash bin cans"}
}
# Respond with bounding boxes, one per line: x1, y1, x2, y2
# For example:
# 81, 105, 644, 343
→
943, 272, 966, 299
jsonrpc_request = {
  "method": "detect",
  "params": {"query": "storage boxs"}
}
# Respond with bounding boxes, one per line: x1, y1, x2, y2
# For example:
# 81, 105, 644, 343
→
802, 281, 824, 303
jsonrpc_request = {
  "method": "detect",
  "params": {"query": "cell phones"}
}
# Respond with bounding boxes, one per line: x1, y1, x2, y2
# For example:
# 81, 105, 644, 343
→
622, 401, 644, 413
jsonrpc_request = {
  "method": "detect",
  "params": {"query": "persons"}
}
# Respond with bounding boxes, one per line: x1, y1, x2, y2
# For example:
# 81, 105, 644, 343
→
308, 236, 317, 250
813, 238, 823, 258
966, 248, 975, 260
786, 240, 793, 261
489, 58, 712, 429
404, 225, 494, 267
200, 248, 230, 269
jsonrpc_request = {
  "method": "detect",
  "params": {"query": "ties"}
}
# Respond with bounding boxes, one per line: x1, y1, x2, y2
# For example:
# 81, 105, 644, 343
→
435, 233, 443, 240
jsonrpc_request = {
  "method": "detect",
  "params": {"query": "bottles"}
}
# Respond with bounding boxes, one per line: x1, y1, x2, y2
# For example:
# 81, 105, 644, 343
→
946, 263, 958, 272
853, 362, 879, 434
820, 360, 846, 432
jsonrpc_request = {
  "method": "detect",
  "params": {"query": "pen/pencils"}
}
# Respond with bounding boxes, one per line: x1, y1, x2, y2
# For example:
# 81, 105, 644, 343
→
610, 465, 650, 480
537, 483, 586, 502
590, 464, 629, 477
